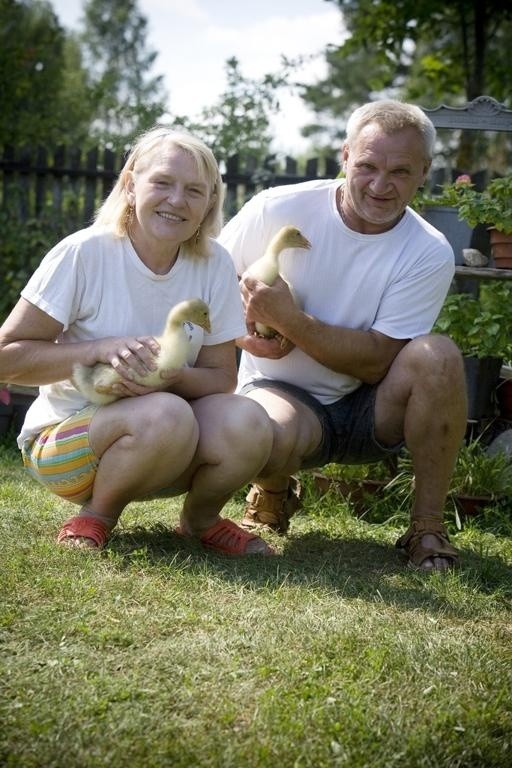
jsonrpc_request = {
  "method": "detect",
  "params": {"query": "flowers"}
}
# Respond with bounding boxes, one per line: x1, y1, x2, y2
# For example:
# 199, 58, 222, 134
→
410, 173, 483, 209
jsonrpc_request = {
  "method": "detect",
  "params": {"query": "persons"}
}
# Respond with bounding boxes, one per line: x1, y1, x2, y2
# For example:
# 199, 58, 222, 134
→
210, 99, 467, 577
0, 123, 275, 557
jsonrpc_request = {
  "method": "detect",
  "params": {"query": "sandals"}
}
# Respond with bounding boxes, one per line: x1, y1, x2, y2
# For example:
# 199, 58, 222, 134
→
396, 514, 460, 576
239, 476, 301, 536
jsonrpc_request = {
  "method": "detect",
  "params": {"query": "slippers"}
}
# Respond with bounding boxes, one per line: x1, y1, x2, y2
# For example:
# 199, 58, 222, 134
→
174, 519, 277, 558
57, 516, 109, 552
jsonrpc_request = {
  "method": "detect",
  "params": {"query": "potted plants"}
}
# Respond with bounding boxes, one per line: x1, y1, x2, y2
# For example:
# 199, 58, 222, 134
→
432, 280, 512, 422
457, 171, 512, 269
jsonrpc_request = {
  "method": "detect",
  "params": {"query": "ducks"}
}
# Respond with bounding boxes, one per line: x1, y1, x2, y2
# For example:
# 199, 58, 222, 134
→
237, 225, 313, 351
70, 297, 214, 408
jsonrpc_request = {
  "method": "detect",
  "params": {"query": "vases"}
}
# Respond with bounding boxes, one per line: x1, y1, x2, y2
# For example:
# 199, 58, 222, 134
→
421, 203, 475, 265
